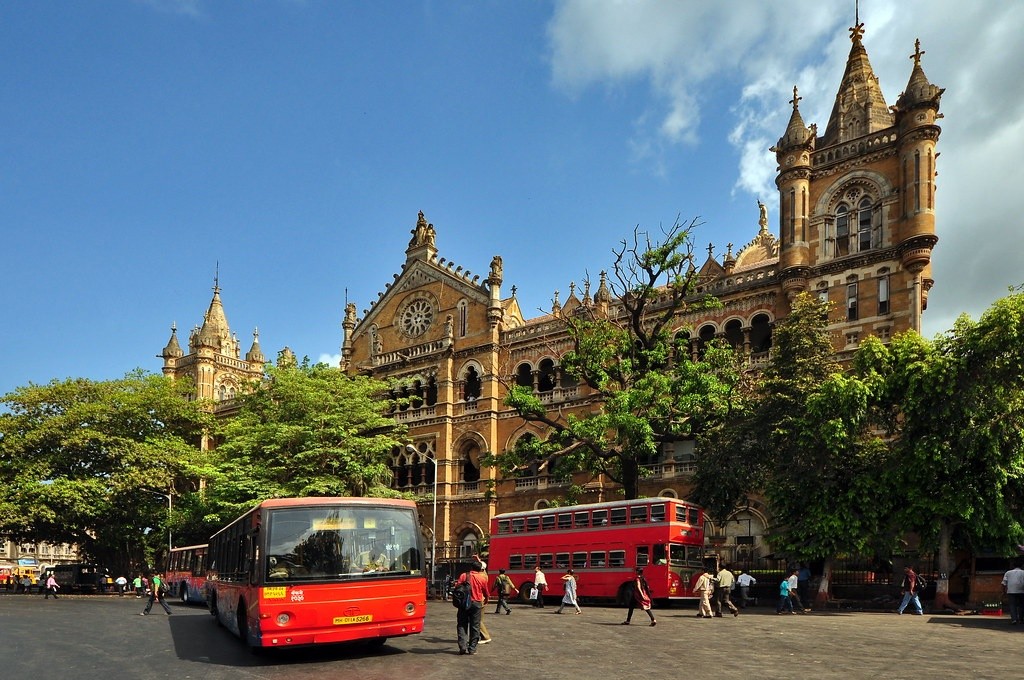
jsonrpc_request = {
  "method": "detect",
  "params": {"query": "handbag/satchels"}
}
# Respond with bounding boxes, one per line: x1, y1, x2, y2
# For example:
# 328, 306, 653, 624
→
530, 588, 538, 599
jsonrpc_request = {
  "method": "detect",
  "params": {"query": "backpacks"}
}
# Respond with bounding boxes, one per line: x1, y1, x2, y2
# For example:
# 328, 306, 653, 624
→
453, 571, 471, 610
915, 574, 928, 591
498, 575, 510, 594
159, 578, 168, 593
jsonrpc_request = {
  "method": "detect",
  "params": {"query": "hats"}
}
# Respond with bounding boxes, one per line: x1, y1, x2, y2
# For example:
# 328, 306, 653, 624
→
479, 561, 486, 569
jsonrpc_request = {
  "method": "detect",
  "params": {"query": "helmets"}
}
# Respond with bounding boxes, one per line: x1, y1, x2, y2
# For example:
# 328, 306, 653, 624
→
567, 569, 574, 574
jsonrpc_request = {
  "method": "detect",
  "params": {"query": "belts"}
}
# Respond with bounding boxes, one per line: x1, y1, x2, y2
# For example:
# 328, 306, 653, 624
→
741, 586, 750, 588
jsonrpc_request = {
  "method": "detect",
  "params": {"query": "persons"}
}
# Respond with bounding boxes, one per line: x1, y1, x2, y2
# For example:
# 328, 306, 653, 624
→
534, 566, 548, 608
757, 200, 767, 220
776, 577, 796, 615
416, 211, 427, 244
554, 568, 582, 615
1001, 563, 1024, 624
444, 552, 491, 655
5, 572, 60, 599
423, 223, 436, 245
891, 565, 923, 615
352, 543, 389, 573
491, 568, 519, 615
115, 571, 173, 616
556, 559, 565, 568
788, 569, 811, 613
620, 568, 656, 627
692, 562, 756, 618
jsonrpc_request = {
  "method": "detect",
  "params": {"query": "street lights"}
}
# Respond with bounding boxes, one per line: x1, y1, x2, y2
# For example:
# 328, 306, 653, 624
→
139, 487, 172, 549
406, 444, 436, 588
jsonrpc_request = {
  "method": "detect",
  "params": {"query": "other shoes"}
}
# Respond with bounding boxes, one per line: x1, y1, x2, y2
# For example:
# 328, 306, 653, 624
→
506, 609, 512, 615
575, 611, 582, 615
649, 622, 656, 627
891, 608, 901, 615
478, 639, 492, 644
164, 612, 172, 615
776, 612, 782, 615
136, 596, 142, 599
912, 613, 921, 615
621, 621, 629, 625
494, 611, 499, 615
715, 613, 722, 617
139, 613, 147, 616
803, 609, 811, 614
697, 614, 712, 618
457, 650, 478, 655
754, 598, 758, 605
790, 611, 797, 615
1011, 619, 1024, 624
730, 608, 738, 617
554, 611, 561, 614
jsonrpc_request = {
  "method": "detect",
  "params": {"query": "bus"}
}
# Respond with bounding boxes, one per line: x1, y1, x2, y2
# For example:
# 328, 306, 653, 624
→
54, 564, 99, 588
166, 543, 209, 604
486, 497, 704, 609
46, 567, 54, 578
205, 496, 431, 647
0, 565, 39, 587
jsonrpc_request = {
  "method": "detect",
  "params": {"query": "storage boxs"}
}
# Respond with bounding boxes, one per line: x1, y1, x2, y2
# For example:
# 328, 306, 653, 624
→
975, 599, 1002, 610
978, 609, 1002, 616
